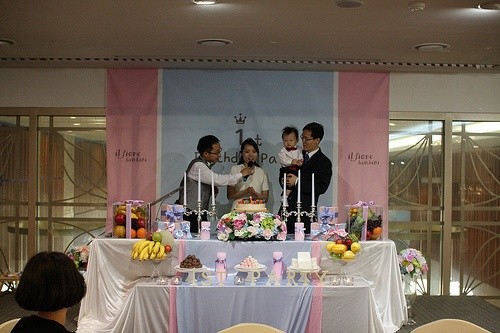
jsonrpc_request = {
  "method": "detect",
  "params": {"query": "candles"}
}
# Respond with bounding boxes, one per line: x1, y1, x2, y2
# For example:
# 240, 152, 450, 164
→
283, 170, 315, 206
184, 169, 216, 204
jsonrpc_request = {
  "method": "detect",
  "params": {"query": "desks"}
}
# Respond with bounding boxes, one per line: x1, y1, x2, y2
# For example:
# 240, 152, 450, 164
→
0, 277, 21, 294
76, 234, 410, 333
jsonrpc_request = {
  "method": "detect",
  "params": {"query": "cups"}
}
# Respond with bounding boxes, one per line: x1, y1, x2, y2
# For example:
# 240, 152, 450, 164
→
156, 275, 168, 285
329, 276, 340, 285
342, 277, 353, 286
171, 276, 182, 284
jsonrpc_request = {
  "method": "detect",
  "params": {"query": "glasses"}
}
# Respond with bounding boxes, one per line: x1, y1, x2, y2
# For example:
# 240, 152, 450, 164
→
210, 148, 222, 154
300, 135, 318, 141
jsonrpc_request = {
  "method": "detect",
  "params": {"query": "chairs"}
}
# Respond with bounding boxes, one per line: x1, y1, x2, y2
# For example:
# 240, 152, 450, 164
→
410, 319, 491, 333
219, 323, 284, 333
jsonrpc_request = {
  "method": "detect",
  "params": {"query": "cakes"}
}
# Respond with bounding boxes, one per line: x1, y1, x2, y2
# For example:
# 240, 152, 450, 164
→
234, 196, 267, 211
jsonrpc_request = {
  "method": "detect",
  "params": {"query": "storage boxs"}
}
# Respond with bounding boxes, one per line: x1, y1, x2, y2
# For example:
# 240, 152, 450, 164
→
152, 204, 192, 238
310, 206, 347, 241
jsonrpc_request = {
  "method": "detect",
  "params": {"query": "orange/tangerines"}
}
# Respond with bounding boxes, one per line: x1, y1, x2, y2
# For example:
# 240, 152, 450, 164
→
373, 227, 382, 235
131, 228, 147, 238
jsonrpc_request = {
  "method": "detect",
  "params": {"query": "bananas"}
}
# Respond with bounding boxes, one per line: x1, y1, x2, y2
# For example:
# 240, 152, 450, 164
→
114, 206, 138, 219
348, 207, 358, 217
131, 239, 165, 261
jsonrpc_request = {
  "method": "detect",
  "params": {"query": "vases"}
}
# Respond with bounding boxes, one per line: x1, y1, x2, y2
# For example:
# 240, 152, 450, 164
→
403, 275, 416, 325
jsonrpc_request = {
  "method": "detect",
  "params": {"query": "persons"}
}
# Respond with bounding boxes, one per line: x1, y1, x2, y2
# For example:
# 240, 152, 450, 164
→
278, 127, 304, 205
175, 135, 252, 233
10, 251, 86, 333
227, 138, 270, 211
277, 122, 332, 234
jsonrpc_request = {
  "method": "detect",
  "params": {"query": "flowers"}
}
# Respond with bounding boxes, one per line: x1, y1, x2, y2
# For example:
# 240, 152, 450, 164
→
397, 248, 429, 276
216, 210, 287, 243
66, 244, 89, 269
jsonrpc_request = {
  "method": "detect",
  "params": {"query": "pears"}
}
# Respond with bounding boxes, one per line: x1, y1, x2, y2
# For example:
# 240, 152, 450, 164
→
326, 242, 361, 261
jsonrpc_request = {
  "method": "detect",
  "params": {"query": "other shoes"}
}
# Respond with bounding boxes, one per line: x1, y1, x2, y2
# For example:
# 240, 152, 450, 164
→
280, 195, 289, 207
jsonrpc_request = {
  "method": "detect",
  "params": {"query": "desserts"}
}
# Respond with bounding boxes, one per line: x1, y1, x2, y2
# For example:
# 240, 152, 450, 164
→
180, 255, 203, 268
239, 255, 259, 268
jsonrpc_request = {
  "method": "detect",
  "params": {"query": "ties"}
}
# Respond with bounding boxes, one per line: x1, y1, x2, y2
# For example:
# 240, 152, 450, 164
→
304, 152, 309, 165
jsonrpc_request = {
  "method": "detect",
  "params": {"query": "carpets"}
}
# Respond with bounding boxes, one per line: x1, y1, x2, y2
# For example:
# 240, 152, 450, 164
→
0, 288, 500, 333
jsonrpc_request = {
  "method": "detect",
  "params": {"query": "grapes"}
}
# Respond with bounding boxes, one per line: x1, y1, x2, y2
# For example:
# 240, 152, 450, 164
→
368, 215, 382, 229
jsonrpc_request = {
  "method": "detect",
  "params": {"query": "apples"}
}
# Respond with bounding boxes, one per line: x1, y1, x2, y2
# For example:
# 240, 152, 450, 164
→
152, 232, 162, 242
367, 230, 378, 240
165, 244, 172, 254
336, 238, 352, 246
114, 215, 145, 237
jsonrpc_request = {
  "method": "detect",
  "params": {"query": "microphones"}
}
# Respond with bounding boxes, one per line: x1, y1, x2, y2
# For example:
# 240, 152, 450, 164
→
243, 161, 253, 182
